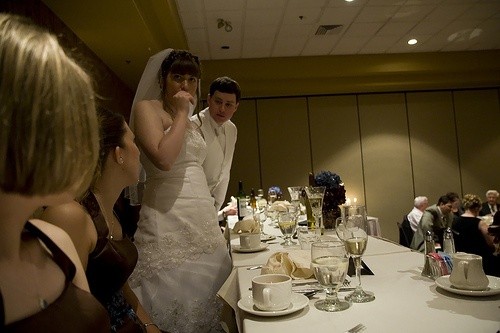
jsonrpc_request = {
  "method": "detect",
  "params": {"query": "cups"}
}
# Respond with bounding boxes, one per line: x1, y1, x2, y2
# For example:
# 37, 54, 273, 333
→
270, 190, 276, 200
288, 186, 303, 216
251, 274, 292, 310
449, 253, 489, 290
240, 233, 261, 249
286, 205, 297, 215
297, 226, 321, 249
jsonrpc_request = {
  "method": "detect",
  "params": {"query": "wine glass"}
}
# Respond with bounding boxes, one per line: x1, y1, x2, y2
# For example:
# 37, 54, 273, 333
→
253, 206, 267, 236
278, 213, 297, 246
311, 241, 350, 312
335, 217, 350, 258
343, 205, 375, 303
305, 186, 326, 243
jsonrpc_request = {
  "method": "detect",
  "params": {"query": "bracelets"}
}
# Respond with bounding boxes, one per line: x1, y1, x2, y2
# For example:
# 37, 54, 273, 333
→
144, 322, 159, 328
223, 210, 226, 218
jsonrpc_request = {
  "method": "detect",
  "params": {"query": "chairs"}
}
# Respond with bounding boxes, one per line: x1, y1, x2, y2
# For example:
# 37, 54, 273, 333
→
437, 228, 461, 252
397, 215, 414, 249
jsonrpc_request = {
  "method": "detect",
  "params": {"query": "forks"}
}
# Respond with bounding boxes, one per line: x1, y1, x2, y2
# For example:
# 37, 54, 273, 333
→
343, 323, 366, 333
343, 279, 351, 284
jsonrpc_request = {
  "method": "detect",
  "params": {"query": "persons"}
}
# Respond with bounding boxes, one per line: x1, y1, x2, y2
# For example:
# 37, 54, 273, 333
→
189, 76, 241, 222
0, 14, 112, 333
126, 48, 233, 333
39, 106, 163, 333
406, 190, 500, 276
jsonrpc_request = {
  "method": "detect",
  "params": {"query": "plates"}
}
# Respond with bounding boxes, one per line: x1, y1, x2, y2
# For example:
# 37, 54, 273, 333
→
292, 272, 351, 283
232, 244, 266, 252
237, 292, 309, 316
436, 275, 500, 295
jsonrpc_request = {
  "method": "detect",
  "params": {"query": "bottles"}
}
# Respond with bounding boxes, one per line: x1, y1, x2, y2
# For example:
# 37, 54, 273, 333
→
443, 229, 455, 253
421, 230, 436, 281
237, 180, 247, 221
250, 187, 254, 206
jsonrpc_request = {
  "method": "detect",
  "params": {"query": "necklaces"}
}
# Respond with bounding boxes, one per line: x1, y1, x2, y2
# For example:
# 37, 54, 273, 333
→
38, 297, 48, 308
110, 235, 114, 240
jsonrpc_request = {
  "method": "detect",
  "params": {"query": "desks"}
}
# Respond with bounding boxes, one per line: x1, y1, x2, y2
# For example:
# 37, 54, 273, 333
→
216, 212, 500, 333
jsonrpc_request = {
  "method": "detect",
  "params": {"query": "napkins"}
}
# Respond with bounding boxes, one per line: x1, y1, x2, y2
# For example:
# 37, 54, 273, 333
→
261, 251, 314, 279
232, 220, 262, 233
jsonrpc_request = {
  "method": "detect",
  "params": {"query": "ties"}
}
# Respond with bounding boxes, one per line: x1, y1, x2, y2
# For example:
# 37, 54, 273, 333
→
492, 205, 495, 213
216, 126, 225, 149
